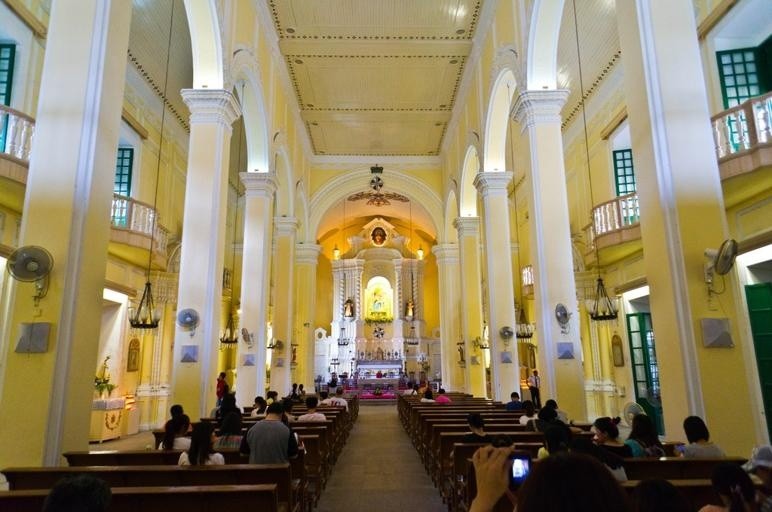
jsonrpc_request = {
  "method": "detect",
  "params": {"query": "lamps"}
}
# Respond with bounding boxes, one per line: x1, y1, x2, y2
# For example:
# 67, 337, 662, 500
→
416, 244, 425, 260
585, 0, 621, 320
338, 198, 349, 346
220, 84, 245, 343
508, 86, 533, 339
407, 199, 418, 347
332, 244, 341, 260
128, 0, 175, 329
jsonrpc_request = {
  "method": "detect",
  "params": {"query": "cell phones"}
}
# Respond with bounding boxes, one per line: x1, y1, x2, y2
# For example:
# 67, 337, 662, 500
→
511, 453, 533, 482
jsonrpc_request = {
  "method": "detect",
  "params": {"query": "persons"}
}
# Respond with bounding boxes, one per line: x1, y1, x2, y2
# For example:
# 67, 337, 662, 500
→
42, 474, 113, 512
157, 372, 349, 480
420, 391, 435, 402
376, 371, 383, 378
526, 370, 541, 409
403, 382, 417, 395
466, 393, 771, 511
418, 381, 428, 394
436, 389, 452, 402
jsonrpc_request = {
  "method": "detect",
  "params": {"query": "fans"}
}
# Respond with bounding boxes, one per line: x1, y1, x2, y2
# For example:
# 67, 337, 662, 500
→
178, 308, 200, 338
268, 338, 279, 350
242, 327, 256, 349
555, 303, 573, 334
7, 244, 55, 306
500, 326, 513, 346
472, 336, 482, 352
703, 239, 738, 297
